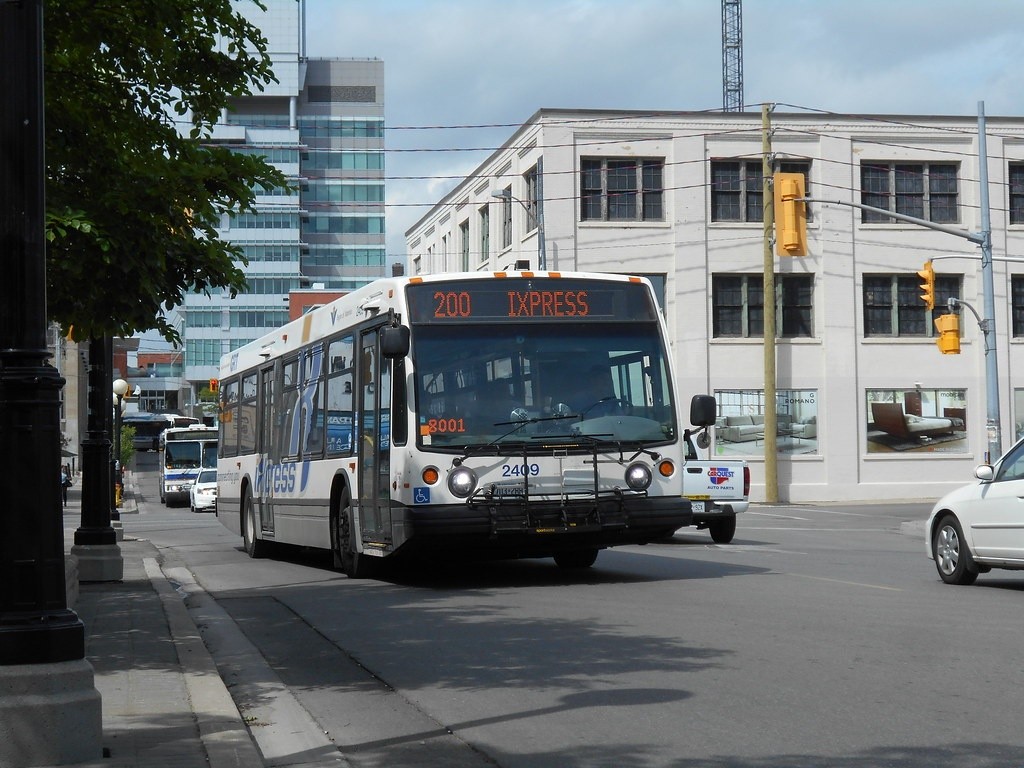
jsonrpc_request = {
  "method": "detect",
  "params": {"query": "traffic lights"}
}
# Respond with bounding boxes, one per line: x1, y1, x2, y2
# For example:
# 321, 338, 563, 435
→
932, 312, 962, 356
919, 260, 936, 313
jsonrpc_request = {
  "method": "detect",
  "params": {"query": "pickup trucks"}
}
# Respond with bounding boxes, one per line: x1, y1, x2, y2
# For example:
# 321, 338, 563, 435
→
680, 433, 751, 545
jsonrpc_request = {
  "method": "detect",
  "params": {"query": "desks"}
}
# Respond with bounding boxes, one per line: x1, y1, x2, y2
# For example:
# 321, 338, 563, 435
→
716, 426, 731, 445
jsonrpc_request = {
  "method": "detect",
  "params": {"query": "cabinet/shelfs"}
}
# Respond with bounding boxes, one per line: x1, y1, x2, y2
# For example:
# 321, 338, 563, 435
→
905, 392, 922, 417
944, 408, 967, 431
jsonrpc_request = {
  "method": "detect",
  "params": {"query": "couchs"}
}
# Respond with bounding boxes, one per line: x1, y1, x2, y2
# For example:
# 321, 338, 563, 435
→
721, 414, 817, 442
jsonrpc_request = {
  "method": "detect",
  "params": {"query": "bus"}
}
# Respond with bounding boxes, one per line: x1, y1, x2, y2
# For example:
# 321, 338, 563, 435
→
109, 411, 200, 453
217, 260, 718, 583
156, 423, 219, 508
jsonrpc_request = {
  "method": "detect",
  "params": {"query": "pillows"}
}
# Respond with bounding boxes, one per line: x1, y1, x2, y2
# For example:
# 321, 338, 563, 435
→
904, 413, 926, 423
799, 415, 816, 424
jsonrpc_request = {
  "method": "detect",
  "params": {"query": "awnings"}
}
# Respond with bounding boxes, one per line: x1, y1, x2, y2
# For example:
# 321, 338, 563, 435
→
61, 450, 78, 457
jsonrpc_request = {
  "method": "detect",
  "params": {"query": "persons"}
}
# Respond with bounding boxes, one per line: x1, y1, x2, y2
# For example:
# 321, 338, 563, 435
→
62, 465, 68, 507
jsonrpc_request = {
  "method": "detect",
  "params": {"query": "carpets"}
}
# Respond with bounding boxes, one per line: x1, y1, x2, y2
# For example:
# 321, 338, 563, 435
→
869, 432, 967, 452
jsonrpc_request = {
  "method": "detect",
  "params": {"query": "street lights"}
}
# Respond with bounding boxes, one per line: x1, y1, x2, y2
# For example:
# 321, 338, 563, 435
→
491, 192, 542, 233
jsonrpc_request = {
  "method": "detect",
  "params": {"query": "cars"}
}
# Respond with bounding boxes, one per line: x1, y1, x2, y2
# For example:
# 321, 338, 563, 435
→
925, 437, 1024, 585
190, 468, 219, 513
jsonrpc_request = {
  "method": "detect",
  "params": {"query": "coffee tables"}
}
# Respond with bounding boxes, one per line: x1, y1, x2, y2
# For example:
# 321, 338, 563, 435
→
754, 430, 804, 446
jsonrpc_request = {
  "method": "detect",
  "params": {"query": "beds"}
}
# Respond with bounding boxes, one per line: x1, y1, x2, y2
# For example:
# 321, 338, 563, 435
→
871, 402, 964, 445
